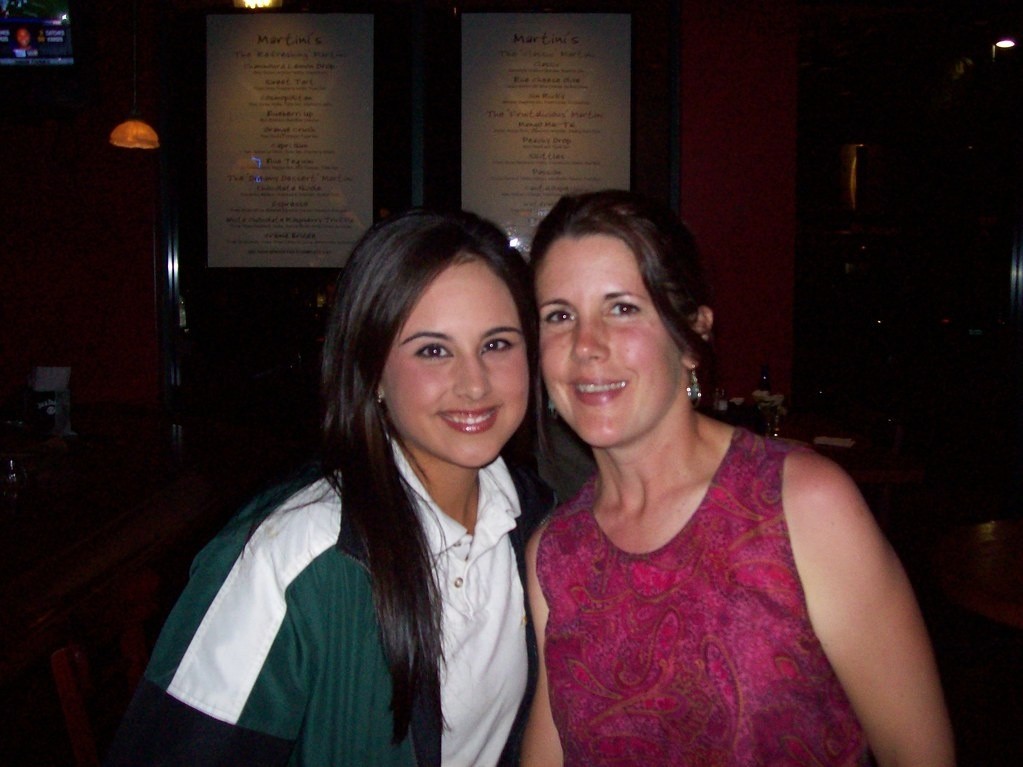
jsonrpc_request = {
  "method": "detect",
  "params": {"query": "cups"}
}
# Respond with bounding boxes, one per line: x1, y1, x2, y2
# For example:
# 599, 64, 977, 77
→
0, 457, 26, 501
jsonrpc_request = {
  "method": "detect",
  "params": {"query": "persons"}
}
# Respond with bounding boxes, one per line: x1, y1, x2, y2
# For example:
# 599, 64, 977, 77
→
115, 189, 955, 767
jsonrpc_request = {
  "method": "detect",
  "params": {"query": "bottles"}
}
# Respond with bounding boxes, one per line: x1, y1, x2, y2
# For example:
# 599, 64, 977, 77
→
757, 362, 771, 395
713, 387, 728, 417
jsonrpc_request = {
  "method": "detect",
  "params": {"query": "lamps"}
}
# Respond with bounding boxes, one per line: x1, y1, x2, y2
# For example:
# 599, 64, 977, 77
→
109, 0, 164, 151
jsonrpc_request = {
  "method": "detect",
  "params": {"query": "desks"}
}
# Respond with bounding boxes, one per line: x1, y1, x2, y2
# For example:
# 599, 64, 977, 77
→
0, 399, 292, 767
926, 517, 1023, 754
